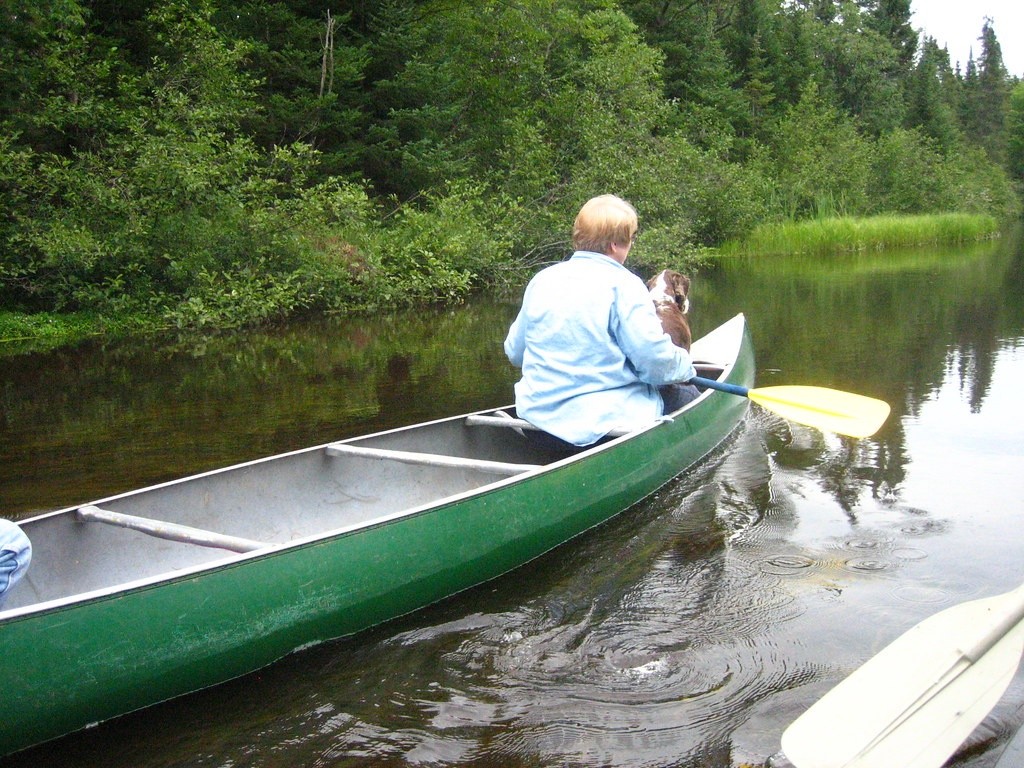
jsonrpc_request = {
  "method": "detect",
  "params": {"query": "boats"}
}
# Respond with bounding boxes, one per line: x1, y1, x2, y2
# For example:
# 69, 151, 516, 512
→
0, 313, 755, 759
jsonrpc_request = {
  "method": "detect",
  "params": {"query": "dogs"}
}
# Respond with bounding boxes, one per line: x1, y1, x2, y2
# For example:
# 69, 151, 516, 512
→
642, 269, 691, 353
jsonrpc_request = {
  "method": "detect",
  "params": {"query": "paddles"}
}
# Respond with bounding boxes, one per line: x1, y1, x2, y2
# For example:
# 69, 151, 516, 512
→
689, 375, 892, 439
779, 579, 1024, 768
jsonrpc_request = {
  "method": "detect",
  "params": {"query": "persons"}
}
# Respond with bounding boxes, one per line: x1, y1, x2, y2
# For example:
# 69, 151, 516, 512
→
504, 192, 702, 447
0, 517, 32, 597
